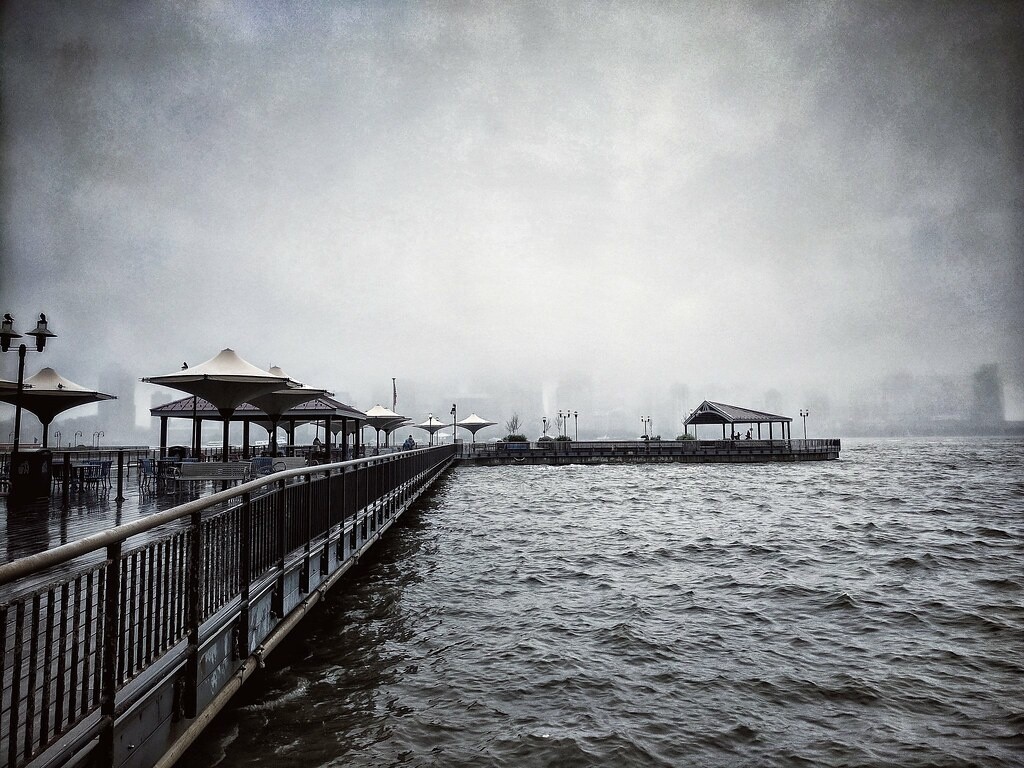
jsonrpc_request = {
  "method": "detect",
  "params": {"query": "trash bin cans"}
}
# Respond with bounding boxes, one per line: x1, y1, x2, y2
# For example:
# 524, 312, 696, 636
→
168, 445, 190, 457
7, 448, 53, 507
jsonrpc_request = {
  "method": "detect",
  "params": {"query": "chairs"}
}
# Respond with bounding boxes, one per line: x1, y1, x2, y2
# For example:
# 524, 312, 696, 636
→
138, 457, 198, 498
52, 460, 113, 494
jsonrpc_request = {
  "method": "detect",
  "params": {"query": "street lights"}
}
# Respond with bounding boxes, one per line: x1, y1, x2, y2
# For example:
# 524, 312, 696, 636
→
0, 312, 58, 451
542, 416, 547, 438
558, 409, 571, 440
573, 411, 579, 441
799, 408, 809, 451
641, 415, 650, 455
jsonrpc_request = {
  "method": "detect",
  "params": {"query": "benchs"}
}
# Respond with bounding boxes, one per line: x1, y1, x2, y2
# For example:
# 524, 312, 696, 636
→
272, 458, 306, 472
173, 461, 253, 495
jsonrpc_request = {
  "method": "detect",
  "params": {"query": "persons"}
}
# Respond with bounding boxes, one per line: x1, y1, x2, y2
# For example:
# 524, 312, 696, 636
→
269, 433, 416, 456
